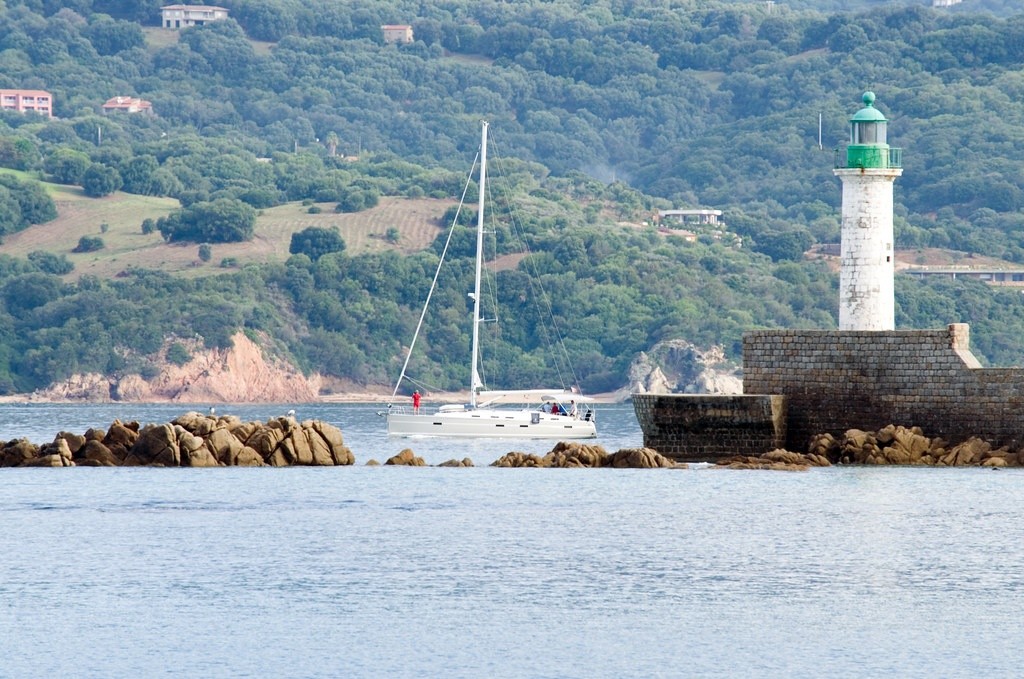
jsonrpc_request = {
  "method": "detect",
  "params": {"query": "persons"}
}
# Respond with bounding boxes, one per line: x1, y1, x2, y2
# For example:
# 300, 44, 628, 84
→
551, 403, 559, 413
412, 390, 421, 415
569, 400, 577, 416
544, 402, 552, 412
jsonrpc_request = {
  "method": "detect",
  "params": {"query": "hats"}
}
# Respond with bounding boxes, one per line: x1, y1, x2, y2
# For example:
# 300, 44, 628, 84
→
415, 390, 419, 392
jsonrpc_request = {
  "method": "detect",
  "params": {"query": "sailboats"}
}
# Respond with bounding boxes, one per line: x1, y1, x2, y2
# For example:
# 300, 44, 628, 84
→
375, 118, 599, 442
